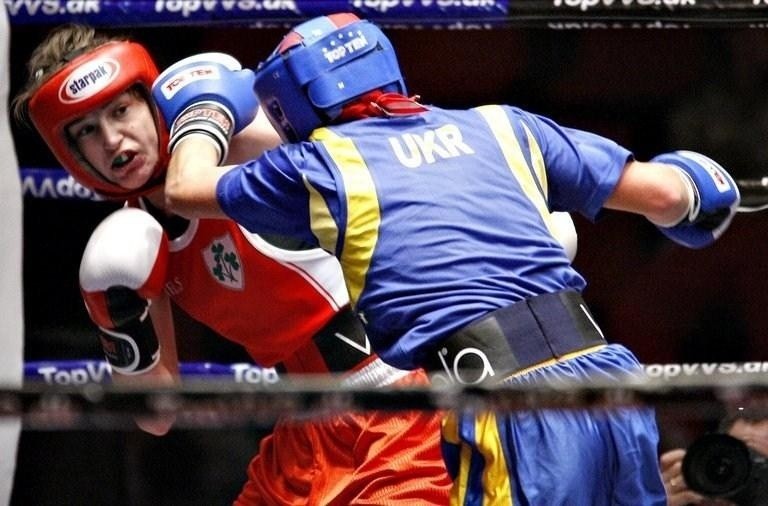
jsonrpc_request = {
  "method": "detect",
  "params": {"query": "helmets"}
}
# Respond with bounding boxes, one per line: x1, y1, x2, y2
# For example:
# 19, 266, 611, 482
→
251, 12, 412, 147
26, 38, 172, 200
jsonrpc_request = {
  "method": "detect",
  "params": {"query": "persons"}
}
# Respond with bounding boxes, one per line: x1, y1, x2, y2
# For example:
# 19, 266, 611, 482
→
147, 9, 745, 506
652, 400, 768, 506
6, 20, 470, 506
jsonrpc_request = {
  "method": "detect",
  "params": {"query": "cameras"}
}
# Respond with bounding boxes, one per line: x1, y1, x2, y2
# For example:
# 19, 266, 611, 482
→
680, 431, 768, 504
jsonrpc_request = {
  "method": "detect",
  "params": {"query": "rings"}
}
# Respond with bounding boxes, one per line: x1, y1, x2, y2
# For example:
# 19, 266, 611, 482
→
669, 479, 678, 490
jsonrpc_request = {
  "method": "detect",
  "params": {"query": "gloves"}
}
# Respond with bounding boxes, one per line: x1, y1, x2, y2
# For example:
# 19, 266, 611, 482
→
645, 144, 743, 253
76, 206, 173, 380
148, 48, 262, 168
